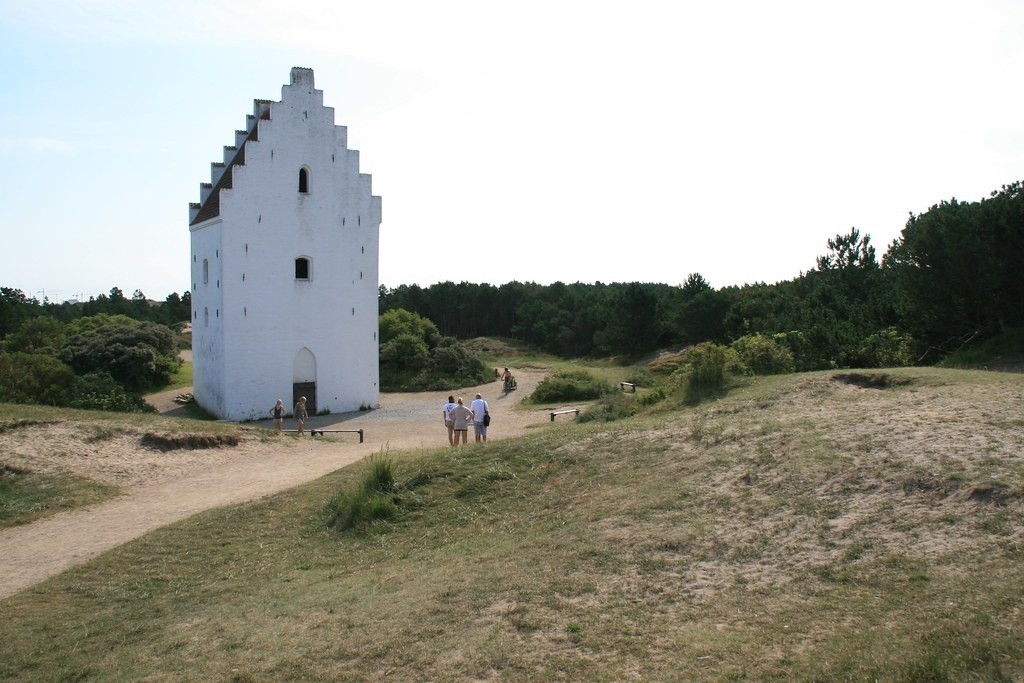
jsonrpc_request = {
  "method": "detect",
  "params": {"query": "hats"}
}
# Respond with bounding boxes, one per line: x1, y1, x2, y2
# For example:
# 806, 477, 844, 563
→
505, 368, 508, 370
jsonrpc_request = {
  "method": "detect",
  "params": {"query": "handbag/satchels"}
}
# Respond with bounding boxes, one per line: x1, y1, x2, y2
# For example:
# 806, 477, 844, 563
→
484, 415, 490, 427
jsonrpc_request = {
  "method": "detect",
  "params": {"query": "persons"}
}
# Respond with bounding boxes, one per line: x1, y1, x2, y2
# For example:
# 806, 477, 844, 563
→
470, 392, 489, 444
292, 396, 309, 435
269, 399, 286, 430
443, 395, 458, 446
448, 397, 474, 446
501, 367, 512, 392
